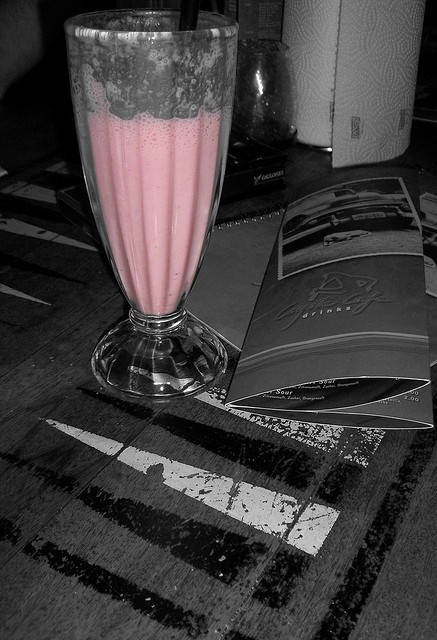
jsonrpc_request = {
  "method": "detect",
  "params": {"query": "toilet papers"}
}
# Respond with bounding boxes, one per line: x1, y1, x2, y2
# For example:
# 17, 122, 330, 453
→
271, 0, 425, 169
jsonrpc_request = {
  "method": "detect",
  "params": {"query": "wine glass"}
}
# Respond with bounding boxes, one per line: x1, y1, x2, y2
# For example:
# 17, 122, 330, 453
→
65, 7, 239, 401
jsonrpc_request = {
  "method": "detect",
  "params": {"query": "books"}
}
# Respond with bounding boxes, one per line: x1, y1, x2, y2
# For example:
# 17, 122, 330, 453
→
183, 204, 287, 354
